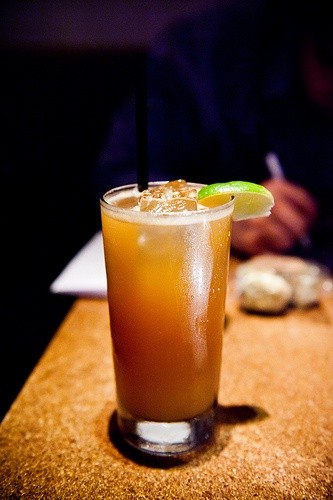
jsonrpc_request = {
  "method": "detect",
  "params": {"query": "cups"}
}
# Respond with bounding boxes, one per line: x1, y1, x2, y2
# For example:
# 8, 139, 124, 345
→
101, 181, 235, 456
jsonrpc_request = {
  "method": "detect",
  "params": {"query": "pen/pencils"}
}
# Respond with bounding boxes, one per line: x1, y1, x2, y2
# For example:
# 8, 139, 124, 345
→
262, 152, 315, 253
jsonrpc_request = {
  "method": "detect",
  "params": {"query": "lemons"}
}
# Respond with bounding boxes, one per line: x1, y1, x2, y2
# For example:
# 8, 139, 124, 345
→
197, 181, 275, 221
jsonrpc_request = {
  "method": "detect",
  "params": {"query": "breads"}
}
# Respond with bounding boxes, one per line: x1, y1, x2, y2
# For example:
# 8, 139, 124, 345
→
233, 253, 322, 312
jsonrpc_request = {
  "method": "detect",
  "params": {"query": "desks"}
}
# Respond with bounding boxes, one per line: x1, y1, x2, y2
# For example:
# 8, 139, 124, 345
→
1, 252, 333, 500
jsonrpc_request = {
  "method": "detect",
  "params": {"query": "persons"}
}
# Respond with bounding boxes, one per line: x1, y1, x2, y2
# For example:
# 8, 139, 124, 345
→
79, 0, 333, 260
